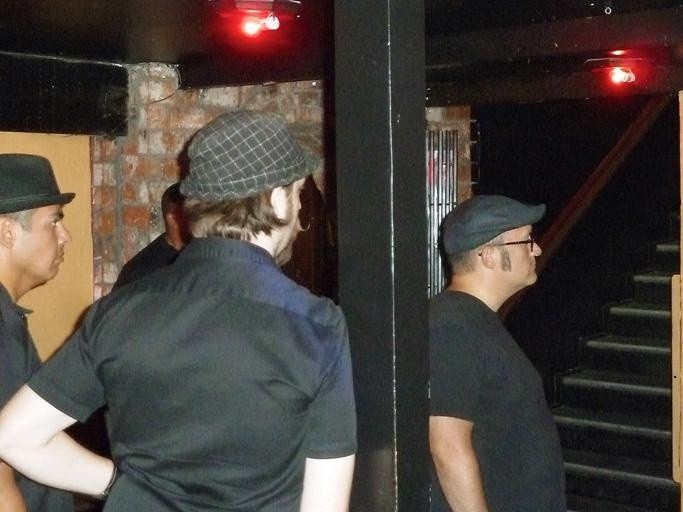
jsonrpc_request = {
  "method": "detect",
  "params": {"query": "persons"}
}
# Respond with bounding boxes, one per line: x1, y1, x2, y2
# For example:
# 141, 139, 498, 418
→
427, 194, 567, 511
0, 153, 77, 512
112, 181, 183, 283
0, 106, 357, 512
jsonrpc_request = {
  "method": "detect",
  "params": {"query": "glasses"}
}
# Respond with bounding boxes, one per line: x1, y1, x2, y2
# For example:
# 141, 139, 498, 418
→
479, 236, 533, 256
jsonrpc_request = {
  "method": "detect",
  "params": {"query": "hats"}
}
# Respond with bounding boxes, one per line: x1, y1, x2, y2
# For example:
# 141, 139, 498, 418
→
181, 110, 319, 200
441, 195, 546, 255
1, 153, 75, 214
